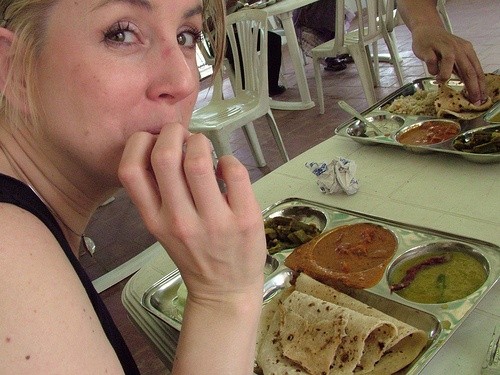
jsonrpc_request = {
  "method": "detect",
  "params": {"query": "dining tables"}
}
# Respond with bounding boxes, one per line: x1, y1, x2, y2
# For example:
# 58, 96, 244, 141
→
122, 136, 499, 375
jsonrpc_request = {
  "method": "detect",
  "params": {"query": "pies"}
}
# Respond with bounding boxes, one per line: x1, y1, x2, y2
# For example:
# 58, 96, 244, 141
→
434, 72, 500, 120
256, 272, 427, 375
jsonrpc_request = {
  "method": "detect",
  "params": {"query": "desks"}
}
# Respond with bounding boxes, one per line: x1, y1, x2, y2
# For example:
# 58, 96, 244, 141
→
245, 0, 319, 112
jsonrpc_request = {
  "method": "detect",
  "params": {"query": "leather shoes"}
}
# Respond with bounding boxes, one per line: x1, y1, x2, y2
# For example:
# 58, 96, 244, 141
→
324, 53, 354, 71
268, 81, 286, 95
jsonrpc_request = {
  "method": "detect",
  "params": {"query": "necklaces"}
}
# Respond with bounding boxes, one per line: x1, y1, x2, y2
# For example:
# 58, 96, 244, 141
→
29, 185, 96, 256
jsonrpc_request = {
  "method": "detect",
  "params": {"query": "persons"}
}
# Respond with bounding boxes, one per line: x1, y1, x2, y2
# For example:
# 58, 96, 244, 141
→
0, 0, 267, 375
203, 0, 286, 97
275, 0, 356, 71
395, 0, 487, 105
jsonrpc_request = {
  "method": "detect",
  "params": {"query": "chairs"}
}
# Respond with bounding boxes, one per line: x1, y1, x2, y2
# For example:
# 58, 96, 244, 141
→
187, 1, 454, 169
